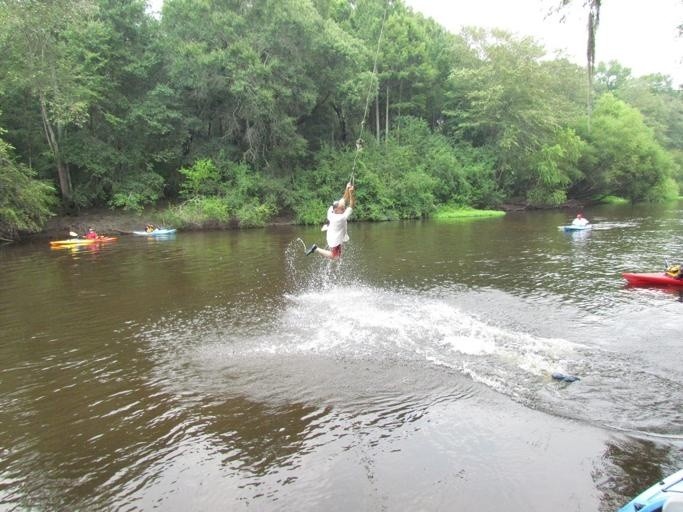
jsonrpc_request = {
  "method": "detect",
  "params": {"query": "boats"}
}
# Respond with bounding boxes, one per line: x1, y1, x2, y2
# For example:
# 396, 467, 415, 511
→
560, 225, 591, 232
49, 235, 117, 246
620, 266, 682, 289
615, 472, 682, 512
112, 227, 177, 238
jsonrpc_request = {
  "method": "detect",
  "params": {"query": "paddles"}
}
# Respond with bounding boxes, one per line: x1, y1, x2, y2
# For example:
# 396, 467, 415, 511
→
69, 231, 83, 238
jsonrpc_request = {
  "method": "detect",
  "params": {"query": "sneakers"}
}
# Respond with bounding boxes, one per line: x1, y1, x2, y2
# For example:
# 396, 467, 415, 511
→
304, 243, 318, 256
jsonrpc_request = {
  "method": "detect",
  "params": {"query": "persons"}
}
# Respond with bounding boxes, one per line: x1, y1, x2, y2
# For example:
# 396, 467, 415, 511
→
81, 228, 99, 239
573, 229, 592, 247
83, 244, 100, 256
571, 214, 589, 226
304, 181, 355, 261
